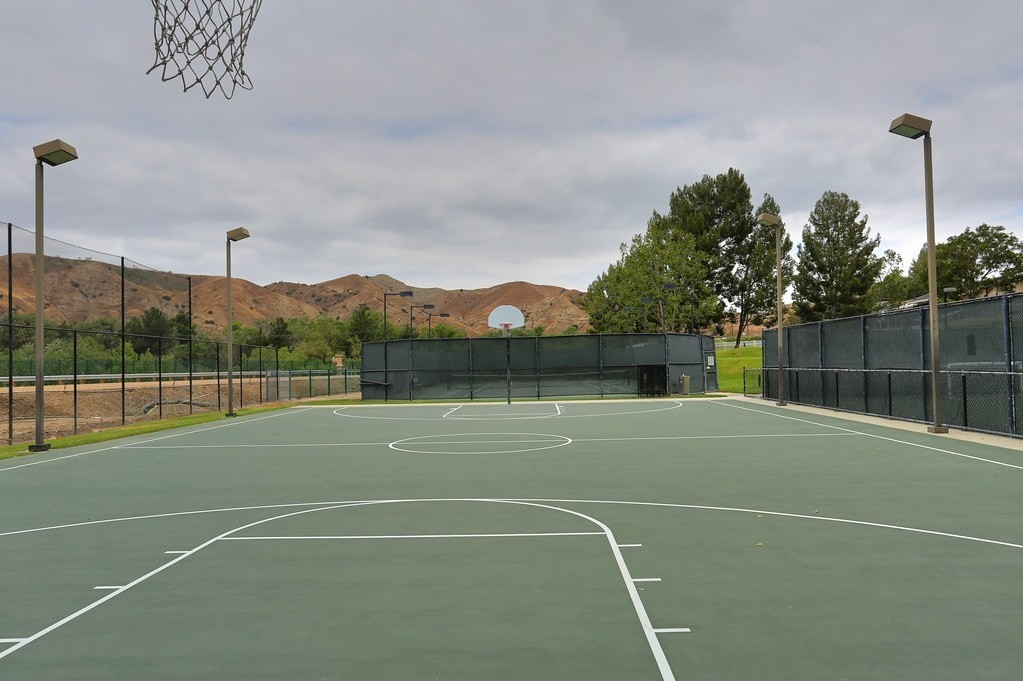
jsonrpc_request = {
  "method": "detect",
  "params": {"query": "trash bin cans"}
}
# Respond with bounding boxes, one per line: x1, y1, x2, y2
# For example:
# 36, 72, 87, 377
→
678, 375, 690, 395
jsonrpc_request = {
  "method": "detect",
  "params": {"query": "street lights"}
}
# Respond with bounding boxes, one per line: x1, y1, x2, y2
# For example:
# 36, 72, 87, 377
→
757, 212, 791, 407
640, 298, 666, 333
889, 113, 949, 433
28, 138, 79, 452
225, 228, 251, 421
408, 305, 435, 400
384, 291, 414, 403
428, 312, 450, 337
662, 282, 696, 335
623, 307, 646, 333
